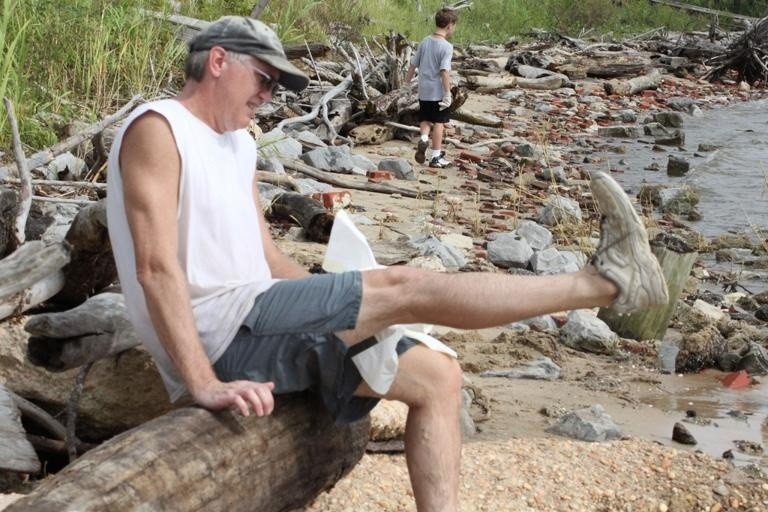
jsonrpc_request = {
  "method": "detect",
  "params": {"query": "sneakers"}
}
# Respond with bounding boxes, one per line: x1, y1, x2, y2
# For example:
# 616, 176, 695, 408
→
429, 151, 453, 168
415, 135, 429, 164
590, 169, 669, 316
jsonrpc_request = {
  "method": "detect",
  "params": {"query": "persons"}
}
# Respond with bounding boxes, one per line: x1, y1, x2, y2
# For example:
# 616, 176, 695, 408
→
405, 9, 458, 168
106, 15, 669, 512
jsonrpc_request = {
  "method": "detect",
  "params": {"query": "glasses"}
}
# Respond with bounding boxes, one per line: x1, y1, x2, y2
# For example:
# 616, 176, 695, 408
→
239, 58, 279, 97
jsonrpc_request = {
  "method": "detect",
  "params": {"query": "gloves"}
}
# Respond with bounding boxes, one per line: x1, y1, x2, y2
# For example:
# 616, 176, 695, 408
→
399, 83, 412, 101
438, 91, 452, 111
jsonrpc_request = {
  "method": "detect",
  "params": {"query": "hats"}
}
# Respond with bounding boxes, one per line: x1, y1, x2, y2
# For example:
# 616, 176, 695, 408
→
189, 15, 310, 94
